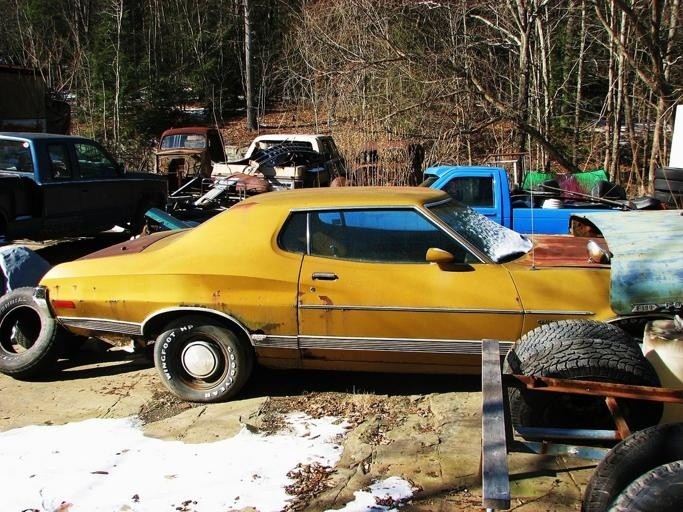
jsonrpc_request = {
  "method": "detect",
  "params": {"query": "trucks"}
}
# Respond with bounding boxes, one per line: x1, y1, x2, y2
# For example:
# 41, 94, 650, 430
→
416, 160, 683, 234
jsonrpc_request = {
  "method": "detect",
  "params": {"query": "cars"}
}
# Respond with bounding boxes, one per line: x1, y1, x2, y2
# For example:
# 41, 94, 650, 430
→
38, 185, 683, 403
155, 127, 226, 180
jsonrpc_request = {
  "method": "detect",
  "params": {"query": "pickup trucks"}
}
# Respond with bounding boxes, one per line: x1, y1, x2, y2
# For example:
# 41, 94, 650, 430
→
0, 132, 168, 239
210, 133, 345, 192
